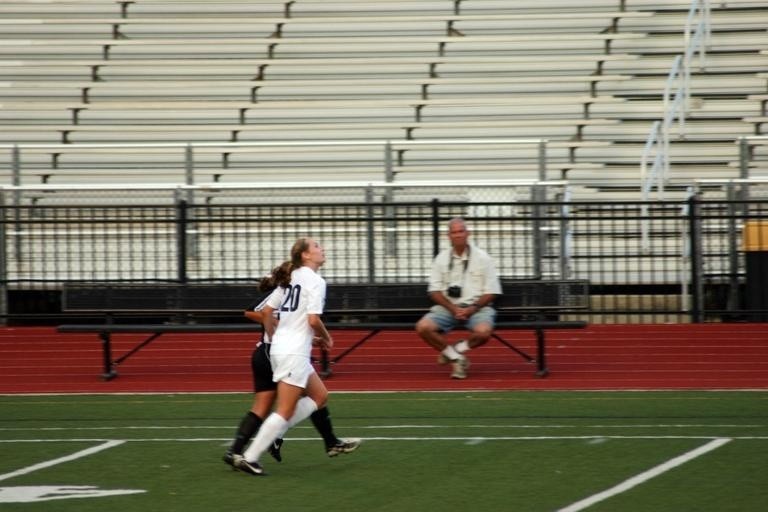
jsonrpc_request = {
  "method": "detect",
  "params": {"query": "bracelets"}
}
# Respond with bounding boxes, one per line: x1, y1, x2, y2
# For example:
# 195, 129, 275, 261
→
471, 304, 481, 308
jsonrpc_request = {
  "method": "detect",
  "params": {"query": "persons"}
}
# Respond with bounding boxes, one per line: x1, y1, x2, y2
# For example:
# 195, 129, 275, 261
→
415, 219, 502, 379
223, 238, 362, 474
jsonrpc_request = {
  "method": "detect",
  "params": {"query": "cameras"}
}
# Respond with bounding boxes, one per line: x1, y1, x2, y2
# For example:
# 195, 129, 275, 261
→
448, 286, 461, 298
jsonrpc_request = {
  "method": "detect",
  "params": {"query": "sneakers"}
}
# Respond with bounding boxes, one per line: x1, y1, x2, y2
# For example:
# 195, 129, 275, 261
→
266, 438, 283, 462
222, 450, 264, 476
437, 339, 470, 379
326, 439, 361, 458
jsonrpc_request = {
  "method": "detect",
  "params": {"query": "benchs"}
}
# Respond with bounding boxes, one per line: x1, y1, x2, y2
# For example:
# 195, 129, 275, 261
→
1, 1, 768, 289
57, 277, 590, 381
